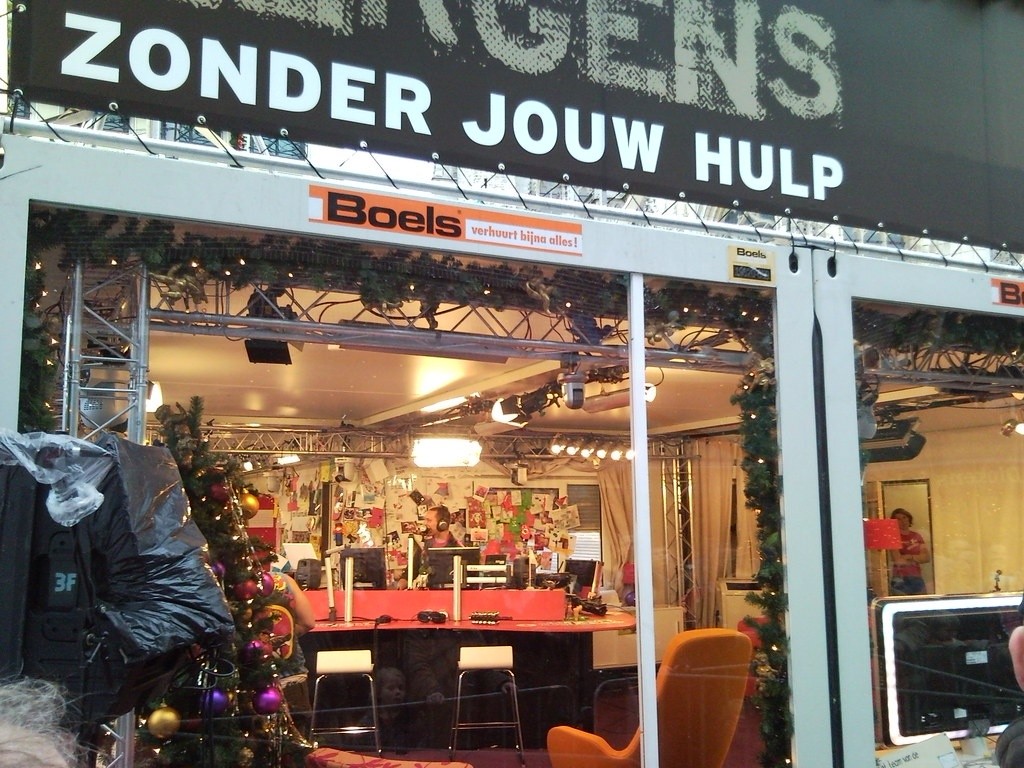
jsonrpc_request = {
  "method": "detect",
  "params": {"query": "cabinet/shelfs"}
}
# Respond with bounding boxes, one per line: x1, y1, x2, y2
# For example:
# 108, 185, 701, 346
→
592, 606, 685, 669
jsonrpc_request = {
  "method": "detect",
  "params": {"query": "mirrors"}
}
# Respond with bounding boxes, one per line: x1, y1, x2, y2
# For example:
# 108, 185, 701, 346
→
881, 477, 936, 596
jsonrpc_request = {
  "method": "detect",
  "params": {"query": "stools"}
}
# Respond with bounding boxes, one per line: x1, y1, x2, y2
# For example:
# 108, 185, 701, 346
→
308, 649, 382, 756
449, 645, 525, 766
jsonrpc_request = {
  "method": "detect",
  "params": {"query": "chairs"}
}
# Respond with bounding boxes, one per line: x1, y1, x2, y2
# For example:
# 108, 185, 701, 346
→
546, 628, 752, 768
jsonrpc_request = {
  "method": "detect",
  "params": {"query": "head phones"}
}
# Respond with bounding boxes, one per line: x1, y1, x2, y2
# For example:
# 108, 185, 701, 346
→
437, 506, 449, 532
573, 597, 607, 616
418, 611, 446, 624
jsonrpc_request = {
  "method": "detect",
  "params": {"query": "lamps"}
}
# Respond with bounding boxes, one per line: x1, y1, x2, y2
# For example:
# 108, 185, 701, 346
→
245, 295, 293, 366
582, 383, 656, 414
549, 434, 636, 461
492, 395, 532, 427
145, 379, 163, 413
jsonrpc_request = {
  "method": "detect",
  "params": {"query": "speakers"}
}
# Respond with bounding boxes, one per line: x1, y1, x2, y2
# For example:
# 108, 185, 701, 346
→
513, 557, 537, 590
296, 559, 322, 589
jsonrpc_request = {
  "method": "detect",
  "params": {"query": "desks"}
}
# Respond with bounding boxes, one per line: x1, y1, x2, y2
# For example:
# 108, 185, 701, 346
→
298, 603, 638, 631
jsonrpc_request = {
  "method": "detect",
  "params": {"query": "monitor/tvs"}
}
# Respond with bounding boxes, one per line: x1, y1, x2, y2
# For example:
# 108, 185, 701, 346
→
341, 548, 386, 591
429, 547, 480, 589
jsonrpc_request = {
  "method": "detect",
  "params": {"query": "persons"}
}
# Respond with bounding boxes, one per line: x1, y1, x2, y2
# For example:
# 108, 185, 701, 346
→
0, 678, 88, 767
996, 594, 1024, 768
397, 505, 479, 591
351, 628, 585, 748
257, 572, 317, 740
894, 611, 990, 733
889, 508, 932, 596
